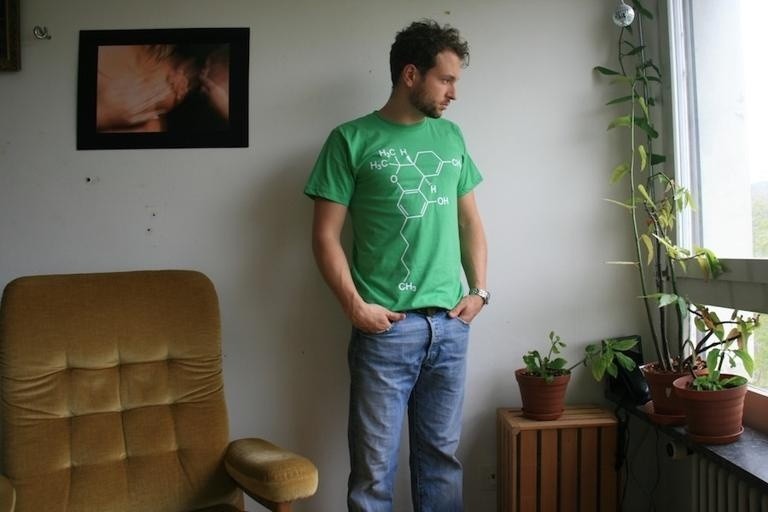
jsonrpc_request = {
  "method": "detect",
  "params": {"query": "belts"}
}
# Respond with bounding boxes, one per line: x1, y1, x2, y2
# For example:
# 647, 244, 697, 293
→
407, 308, 447, 315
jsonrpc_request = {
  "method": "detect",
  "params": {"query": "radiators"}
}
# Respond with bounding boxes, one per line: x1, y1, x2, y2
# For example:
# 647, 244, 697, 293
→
691, 449, 768, 512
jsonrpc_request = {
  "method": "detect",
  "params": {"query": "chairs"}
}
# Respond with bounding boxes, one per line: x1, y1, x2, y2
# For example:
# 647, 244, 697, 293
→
0, 268, 320, 512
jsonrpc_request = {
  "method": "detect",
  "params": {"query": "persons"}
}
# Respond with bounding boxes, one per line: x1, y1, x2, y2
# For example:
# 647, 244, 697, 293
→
303, 20, 492, 511
96, 40, 233, 133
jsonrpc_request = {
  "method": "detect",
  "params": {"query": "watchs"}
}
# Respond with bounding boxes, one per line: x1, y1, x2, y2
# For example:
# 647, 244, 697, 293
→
469, 287, 491, 305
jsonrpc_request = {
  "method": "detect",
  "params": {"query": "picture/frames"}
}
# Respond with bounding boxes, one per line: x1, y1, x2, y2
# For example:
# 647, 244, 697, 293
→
76, 27, 250, 150
